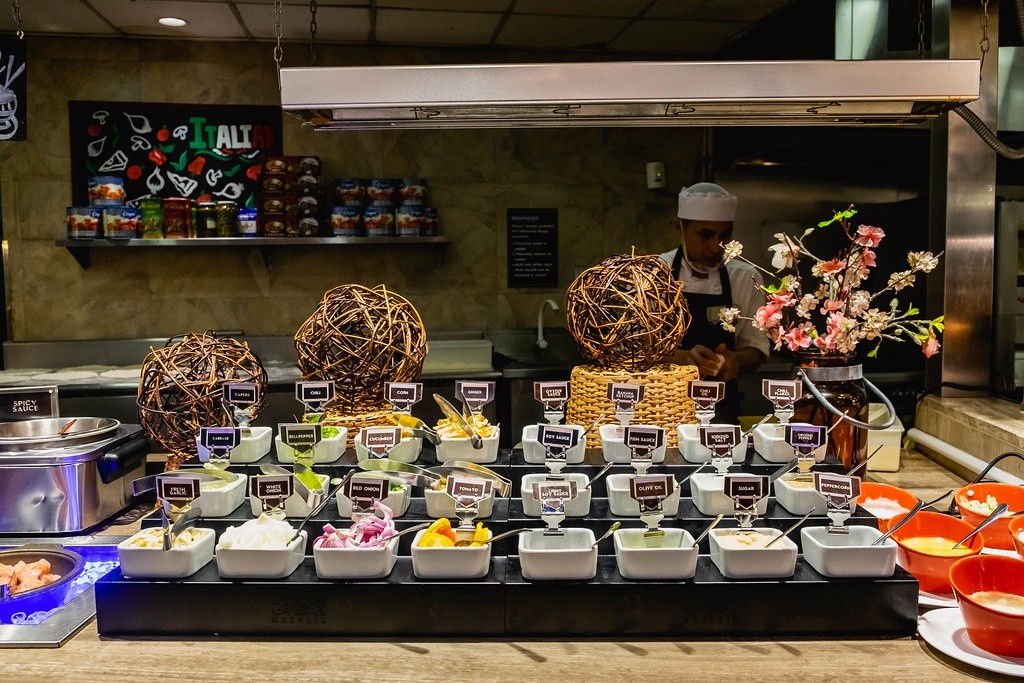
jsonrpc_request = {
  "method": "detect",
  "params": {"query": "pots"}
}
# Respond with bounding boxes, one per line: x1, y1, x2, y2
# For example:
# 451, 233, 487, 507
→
0, 417, 122, 453
0, 542, 86, 624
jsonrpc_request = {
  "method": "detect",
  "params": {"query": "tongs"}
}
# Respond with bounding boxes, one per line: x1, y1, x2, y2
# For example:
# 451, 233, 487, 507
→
357, 458, 442, 490
159, 507, 202, 551
260, 463, 325, 509
130, 468, 239, 496
426, 461, 512, 498
433, 391, 483, 450
413, 419, 442, 446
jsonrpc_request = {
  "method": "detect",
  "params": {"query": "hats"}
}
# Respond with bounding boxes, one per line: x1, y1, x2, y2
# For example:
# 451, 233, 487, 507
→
677, 182, 738, 221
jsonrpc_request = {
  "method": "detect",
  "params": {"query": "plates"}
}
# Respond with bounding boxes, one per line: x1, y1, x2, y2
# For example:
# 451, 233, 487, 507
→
917, 608, 1024, 678
918, 590, 960, 608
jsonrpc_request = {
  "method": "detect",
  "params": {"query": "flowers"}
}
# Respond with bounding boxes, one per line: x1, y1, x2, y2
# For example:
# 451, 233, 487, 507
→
717, 202, 946, 358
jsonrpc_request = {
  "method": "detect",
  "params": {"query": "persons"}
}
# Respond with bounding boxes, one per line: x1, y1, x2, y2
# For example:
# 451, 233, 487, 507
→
623, 183, 770, 424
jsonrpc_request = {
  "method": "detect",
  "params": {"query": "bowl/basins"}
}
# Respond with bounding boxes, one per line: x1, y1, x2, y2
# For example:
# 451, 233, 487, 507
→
1008, 513, 1024, 561
887, 510, 984, 594
955, 482, 1024, 551
857, 482, 919, 534
948, 555, 1024, 659
115, 420, 899, 577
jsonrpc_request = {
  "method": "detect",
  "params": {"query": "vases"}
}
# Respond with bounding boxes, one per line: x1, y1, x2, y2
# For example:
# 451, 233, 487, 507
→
800, 366, 869, 480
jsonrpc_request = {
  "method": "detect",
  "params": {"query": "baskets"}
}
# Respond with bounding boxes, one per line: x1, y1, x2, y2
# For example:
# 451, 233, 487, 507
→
156, 454, 191, 511
565, 364, 701, 448
303, 403, 401, 448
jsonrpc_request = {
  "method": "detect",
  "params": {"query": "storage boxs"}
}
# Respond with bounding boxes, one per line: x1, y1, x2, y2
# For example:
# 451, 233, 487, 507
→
421, 340, 493, 372
709, 527, 797, 578
519, 528, 598, 581
800, 525, 897, 576
216, 529, 307, 579
614, 528, 699, 579
163, 415, 904, 518
411, 528, 492, 578
314, 529, 400, 579
117, 526, 215, 578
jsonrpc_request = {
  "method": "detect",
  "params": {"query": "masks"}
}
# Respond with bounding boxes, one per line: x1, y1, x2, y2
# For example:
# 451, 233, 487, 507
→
679, 222, 724, 273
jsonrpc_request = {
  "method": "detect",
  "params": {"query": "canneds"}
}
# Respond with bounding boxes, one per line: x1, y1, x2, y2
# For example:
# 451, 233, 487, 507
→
328, 177, 439, 237
65, 174, 139, 240
196, 201, 239, 237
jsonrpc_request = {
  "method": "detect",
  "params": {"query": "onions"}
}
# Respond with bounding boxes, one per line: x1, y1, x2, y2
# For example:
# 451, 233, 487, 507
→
313, 502, 395, 550
